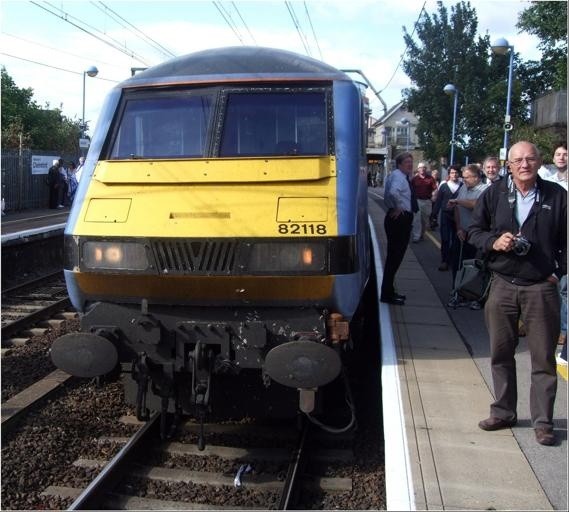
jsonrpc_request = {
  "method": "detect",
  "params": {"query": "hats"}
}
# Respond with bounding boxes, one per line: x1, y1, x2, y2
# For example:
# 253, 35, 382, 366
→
418, 162, 427, 168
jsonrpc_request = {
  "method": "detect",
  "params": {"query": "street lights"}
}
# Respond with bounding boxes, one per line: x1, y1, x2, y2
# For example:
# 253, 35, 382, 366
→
490, 36, 517, 171
80, 64, 101, 158
401, 117, 412, 153
441, 83, 460, 170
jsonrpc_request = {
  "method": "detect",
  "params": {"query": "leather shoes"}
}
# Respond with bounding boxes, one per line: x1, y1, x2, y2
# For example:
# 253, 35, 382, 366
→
381, 297, 404, 305
518, 327, 525, 336
558, 335, 566, 345
395, 293, 406, 300
479, 417, 507, 430
535, 428, 554, 445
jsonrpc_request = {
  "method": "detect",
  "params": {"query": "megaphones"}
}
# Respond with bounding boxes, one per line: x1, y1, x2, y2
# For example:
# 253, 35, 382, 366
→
504, 123, 513, 130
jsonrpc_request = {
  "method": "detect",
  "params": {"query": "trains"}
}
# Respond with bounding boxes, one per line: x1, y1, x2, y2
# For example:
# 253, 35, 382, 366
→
47, 45, 375, 434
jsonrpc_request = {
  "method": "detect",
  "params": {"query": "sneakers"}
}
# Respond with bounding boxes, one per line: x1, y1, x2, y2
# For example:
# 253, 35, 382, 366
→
470, 301, 481, 310
412, 236, 419, 242
439, 263, 448, 271
448, 300, 469, 307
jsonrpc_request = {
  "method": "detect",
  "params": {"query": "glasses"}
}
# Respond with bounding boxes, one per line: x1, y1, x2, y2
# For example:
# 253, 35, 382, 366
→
508, 157, 538, 166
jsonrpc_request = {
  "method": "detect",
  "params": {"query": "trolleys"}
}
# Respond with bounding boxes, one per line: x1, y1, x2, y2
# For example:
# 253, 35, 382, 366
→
449, 232, 484, 311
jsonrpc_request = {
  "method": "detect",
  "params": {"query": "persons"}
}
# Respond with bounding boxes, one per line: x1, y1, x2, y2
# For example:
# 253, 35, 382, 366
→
47, 156, 87, 209
468, 139, 568, 445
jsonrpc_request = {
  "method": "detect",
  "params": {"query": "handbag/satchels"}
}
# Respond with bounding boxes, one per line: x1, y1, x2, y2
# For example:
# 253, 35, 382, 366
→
452, 259, 490, 305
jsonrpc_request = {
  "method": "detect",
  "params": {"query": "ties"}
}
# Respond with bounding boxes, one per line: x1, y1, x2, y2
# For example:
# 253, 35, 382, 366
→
407, 175, 419, 213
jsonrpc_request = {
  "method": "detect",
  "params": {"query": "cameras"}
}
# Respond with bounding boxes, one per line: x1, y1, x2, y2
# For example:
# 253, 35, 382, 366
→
510, 236, 531, 256
